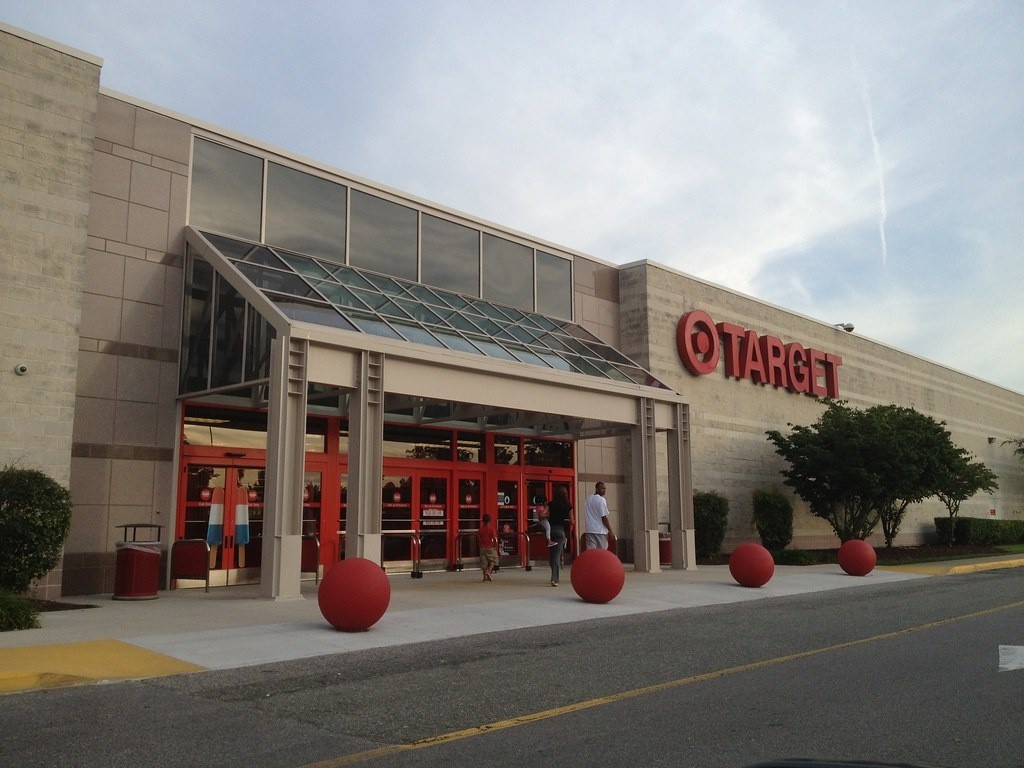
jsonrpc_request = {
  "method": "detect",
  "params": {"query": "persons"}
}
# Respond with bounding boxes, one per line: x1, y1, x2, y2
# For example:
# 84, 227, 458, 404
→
538, 485, 575, 587
584, 482, 618, 549
476, 514, 498, 582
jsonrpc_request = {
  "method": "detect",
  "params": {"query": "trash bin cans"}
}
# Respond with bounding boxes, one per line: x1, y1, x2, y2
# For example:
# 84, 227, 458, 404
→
112, 522, 164, 600
658, 521, 672, 565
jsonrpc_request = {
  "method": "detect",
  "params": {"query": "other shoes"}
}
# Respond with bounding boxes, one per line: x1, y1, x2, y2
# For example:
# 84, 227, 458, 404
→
552, 580, 559, 587
483, 573, 493, 582
546, 542, 558, 548
